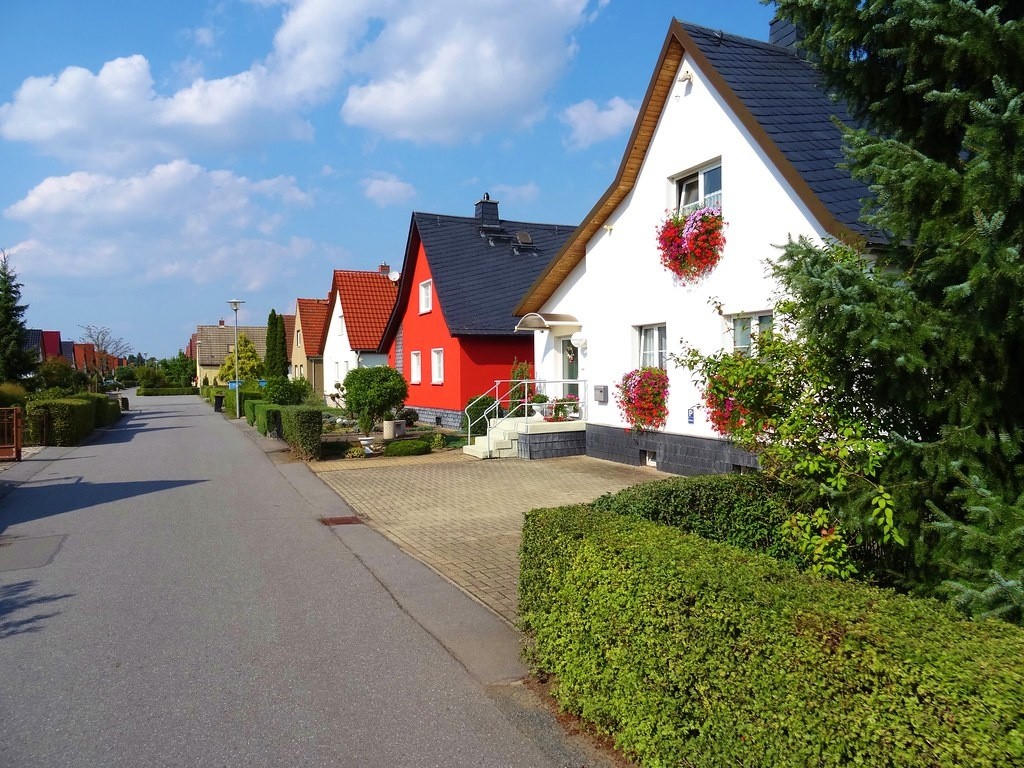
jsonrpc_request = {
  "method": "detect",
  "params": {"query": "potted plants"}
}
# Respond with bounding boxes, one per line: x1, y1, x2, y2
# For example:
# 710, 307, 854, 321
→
358, 407, 375, 453
532, 395, 548, 418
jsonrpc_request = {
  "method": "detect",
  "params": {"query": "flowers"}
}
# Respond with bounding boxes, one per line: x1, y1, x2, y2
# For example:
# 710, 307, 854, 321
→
518, 397, 533, 410
613, 367, 669, 439
700, 376, 768, 439
655, 203, 728, 286
542, 395, 580, 422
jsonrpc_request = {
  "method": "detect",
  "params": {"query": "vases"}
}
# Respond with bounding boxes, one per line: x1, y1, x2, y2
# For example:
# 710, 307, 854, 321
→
560, 405, 574, 421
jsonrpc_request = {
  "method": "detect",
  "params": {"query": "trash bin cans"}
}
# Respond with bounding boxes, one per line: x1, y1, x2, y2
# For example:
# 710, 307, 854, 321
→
214, 395, 225, 412
122, 398, 129, 411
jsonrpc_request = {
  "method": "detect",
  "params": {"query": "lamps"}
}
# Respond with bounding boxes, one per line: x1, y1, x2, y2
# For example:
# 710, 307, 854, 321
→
571, 330, 588, 349
713, 29, 723, 47
677, 71, 692, 85
